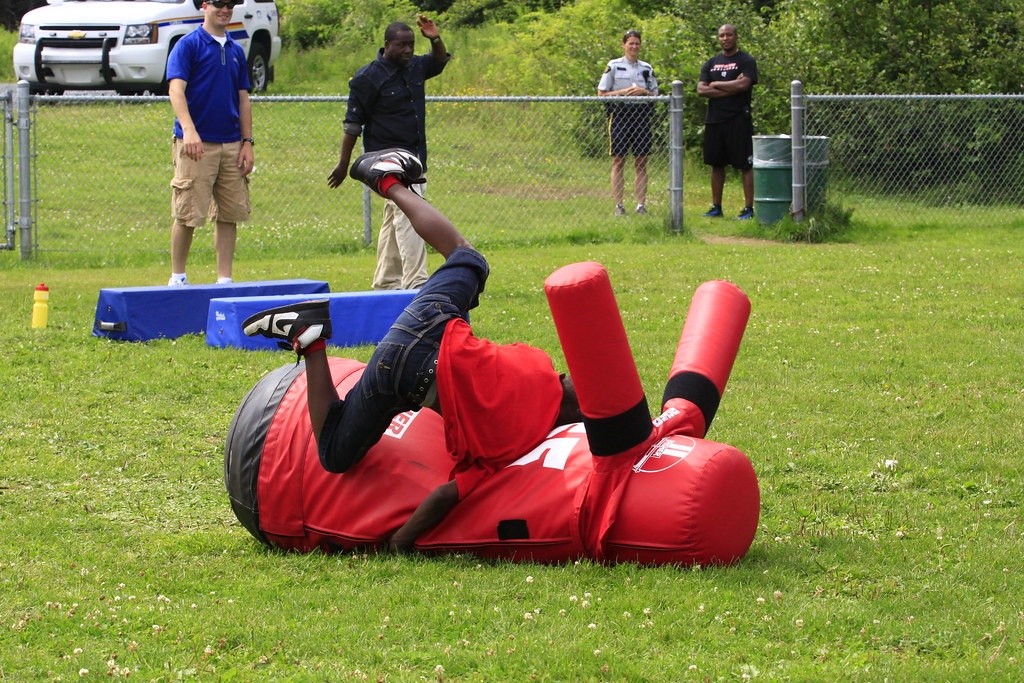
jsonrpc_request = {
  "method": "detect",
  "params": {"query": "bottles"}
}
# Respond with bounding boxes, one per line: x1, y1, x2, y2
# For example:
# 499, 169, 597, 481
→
30, 283, 50, 329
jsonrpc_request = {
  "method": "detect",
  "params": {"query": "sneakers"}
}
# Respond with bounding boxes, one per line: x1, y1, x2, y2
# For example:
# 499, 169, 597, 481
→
168, 278, 189, 286
636, 207, 648, 214
242, 298, 333, 358
736, 210, 753, 221
352, 147, 424, 196
704, 207, 725, 218
615, 208, 626, 215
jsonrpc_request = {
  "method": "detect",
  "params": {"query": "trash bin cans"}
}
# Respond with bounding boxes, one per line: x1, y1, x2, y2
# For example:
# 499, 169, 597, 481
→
752, 133, 830, 227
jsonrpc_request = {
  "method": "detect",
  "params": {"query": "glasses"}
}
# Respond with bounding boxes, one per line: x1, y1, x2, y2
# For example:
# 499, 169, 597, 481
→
204, 1, 237, 9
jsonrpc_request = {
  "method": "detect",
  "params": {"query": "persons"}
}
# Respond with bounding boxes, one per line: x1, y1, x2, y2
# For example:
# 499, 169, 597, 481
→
327, 14, 450, 291
165, 1, 255, 286
244, 148, 583, 559
697, 24, 759, 221
597, 32, 660, 216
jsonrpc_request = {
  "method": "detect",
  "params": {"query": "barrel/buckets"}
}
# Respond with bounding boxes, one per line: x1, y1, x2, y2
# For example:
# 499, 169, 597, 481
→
752, 133, 831, 226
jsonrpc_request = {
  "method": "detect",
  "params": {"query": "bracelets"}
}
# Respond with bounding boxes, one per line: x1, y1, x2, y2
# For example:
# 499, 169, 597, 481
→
242, 137, 254, 146
430, 36, 441, 44
644, 90, 650, 96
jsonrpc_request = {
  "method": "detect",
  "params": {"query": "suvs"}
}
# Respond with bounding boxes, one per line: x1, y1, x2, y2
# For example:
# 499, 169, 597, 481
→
12, 1, 283, 97
